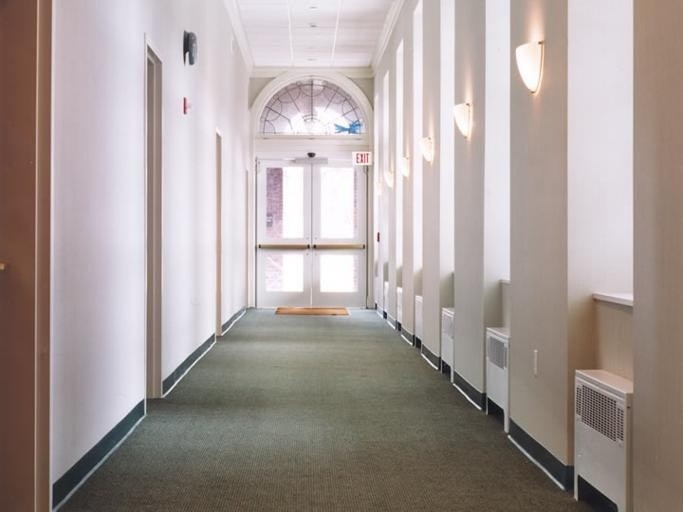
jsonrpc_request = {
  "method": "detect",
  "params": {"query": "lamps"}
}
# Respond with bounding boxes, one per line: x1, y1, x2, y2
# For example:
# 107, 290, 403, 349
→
515, 40, 546, 94
453, 102, 472, 138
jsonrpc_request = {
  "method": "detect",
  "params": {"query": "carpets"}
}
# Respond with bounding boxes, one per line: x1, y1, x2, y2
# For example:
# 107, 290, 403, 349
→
274, 306, 349, 315
